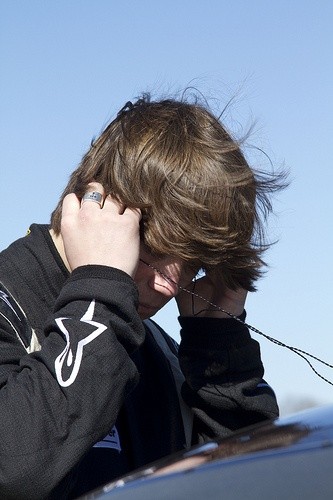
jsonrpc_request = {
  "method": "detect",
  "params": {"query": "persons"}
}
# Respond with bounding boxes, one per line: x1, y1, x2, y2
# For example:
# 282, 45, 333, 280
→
0, 86, 292, 500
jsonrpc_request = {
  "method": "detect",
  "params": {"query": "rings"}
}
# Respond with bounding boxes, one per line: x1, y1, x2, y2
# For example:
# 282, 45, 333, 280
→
81, 191, 105, 209
